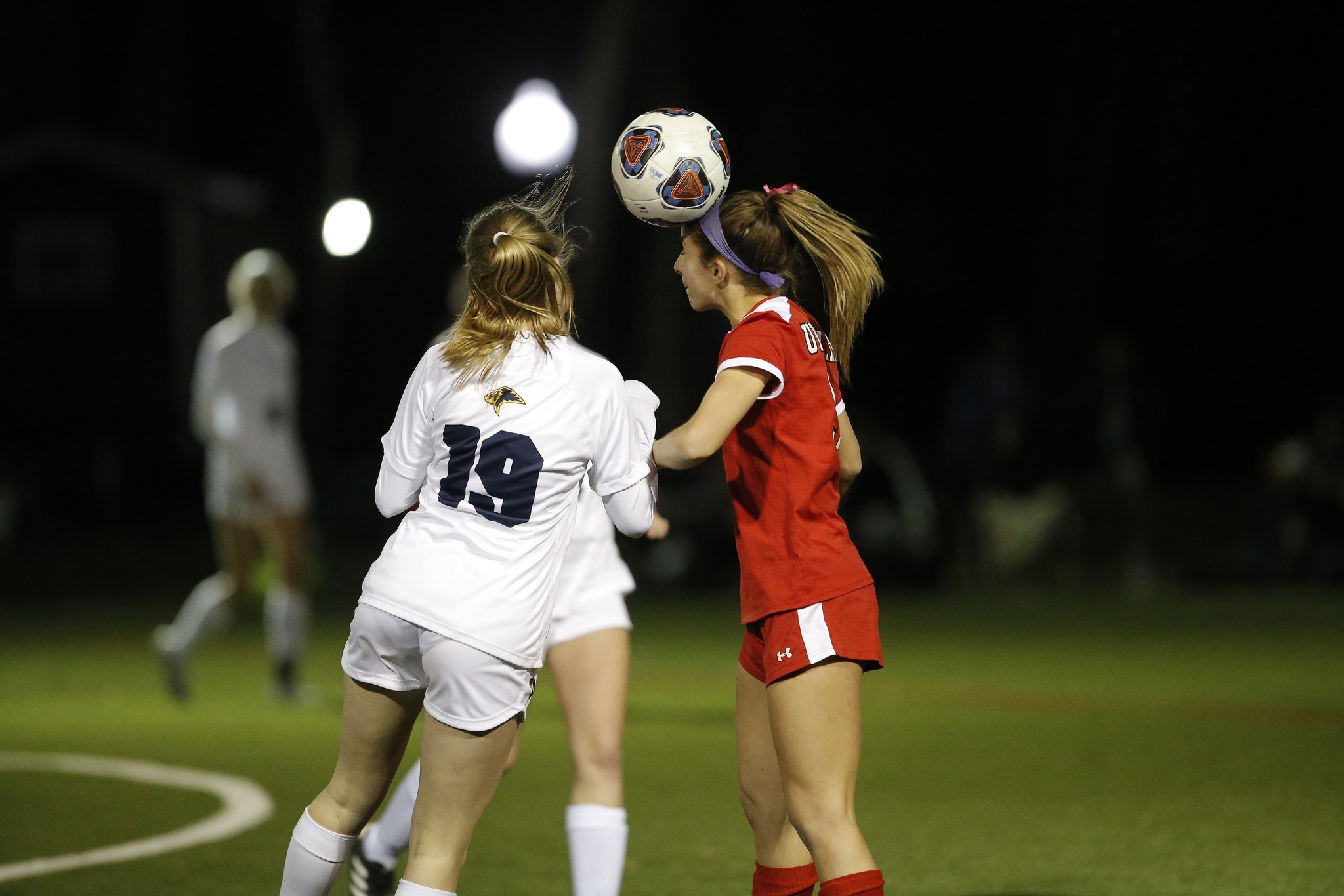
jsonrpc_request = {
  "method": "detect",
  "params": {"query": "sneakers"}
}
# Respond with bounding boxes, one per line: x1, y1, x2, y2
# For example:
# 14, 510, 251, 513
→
348, 821, 397, 896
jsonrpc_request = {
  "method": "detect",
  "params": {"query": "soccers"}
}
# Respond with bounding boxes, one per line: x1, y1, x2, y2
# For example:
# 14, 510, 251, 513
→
610, 108, 731, 228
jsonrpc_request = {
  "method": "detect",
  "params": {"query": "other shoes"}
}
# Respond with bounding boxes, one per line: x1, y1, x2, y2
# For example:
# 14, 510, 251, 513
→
148, 621, 190, 704
265, 647, 296, 702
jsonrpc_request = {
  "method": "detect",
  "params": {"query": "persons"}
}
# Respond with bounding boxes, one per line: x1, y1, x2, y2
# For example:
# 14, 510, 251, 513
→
148, 248, 317, 705
964, 406, 1071, 590
351, 456, 668, 895
279, 163, 657, 896
651, 182, 885, 896
851, 422, 937, 576
